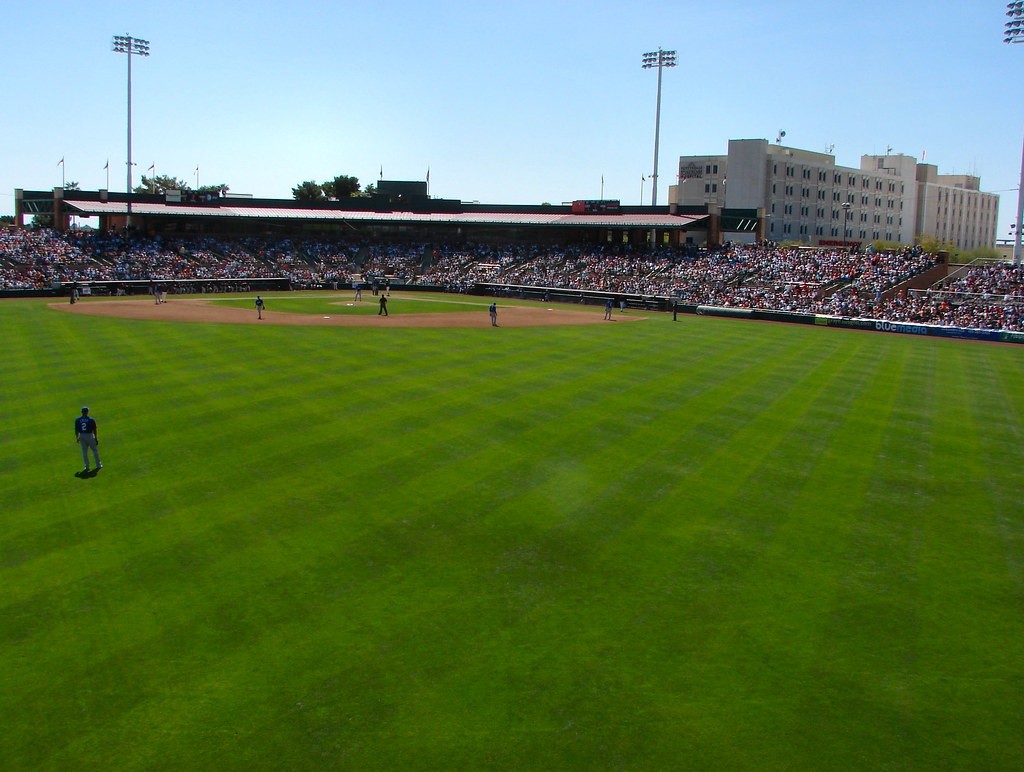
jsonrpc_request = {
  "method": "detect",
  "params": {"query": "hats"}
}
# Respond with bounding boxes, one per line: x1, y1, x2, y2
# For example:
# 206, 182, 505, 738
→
82, 407, 88, 412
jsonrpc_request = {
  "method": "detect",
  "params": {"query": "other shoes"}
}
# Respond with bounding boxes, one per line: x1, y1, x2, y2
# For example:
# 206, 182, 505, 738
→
85, 467, 90, 472
98, 465, 103, 468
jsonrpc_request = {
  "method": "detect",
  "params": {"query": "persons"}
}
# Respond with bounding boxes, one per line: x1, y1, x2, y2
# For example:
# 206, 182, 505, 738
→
0, 220, 1024, 332
74, 408, 102, 472
